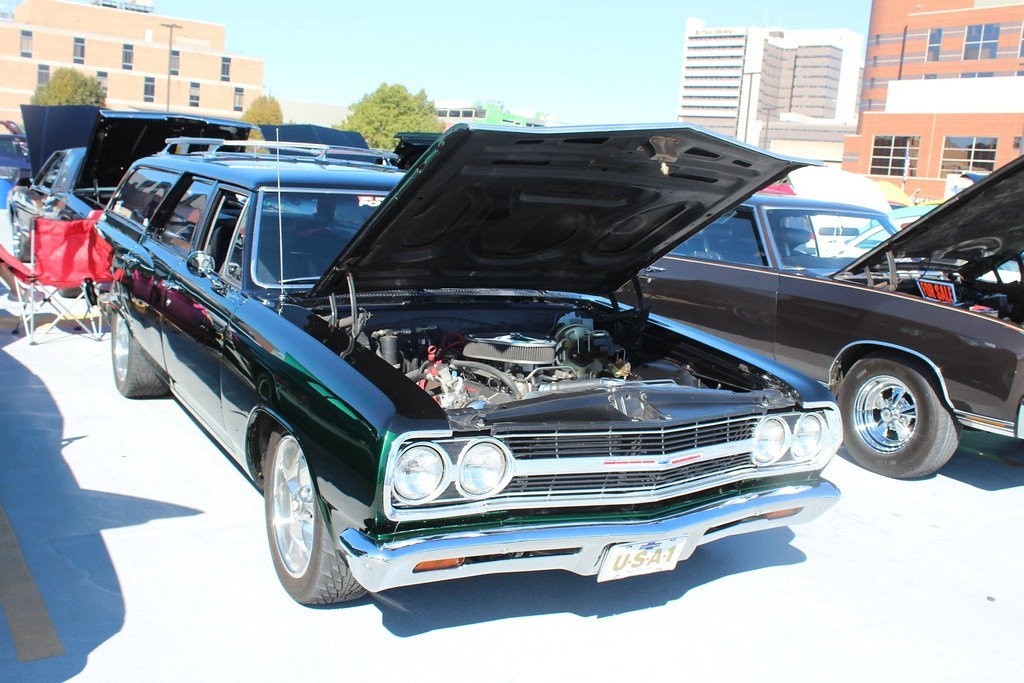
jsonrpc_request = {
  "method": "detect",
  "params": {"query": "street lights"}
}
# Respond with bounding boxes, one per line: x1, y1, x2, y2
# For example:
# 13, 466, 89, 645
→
160, 22, 183, 113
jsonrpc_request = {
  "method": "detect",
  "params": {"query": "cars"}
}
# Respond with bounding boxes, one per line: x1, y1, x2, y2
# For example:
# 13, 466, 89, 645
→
7, 108, 1023, 607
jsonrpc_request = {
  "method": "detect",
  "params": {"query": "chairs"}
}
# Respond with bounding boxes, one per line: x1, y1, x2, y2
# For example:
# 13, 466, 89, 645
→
209, 223, 272, 278
0, 210, 125, 346
775, 226, 810, 257
